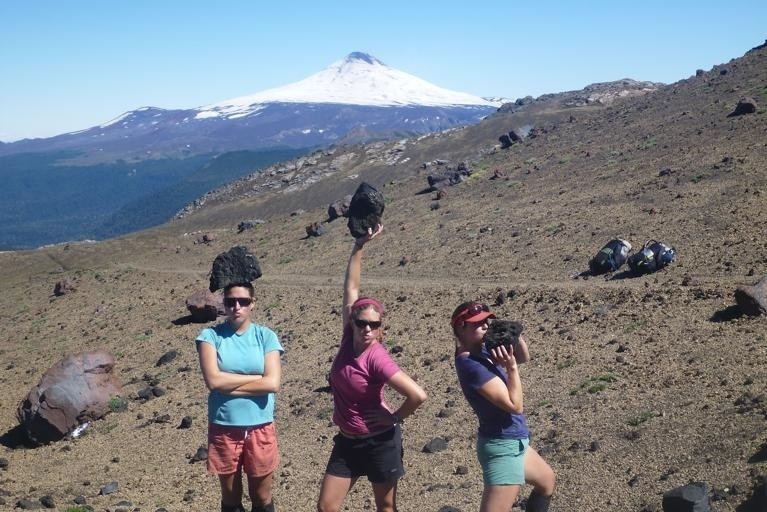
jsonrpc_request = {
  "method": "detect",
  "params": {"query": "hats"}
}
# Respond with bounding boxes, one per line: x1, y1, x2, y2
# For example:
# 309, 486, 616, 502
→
449, 302, 497, 329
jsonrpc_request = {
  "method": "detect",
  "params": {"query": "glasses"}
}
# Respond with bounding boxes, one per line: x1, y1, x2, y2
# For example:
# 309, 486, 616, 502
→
222, 298, 251, 308
453, 304, 489, 326
352, 314, 382, 329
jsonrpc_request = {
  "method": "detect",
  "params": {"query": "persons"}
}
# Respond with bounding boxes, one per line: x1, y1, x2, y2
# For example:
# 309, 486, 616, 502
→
316, 220, 426, 511
446, 300, 557, 510
195, 278, 285, 510
